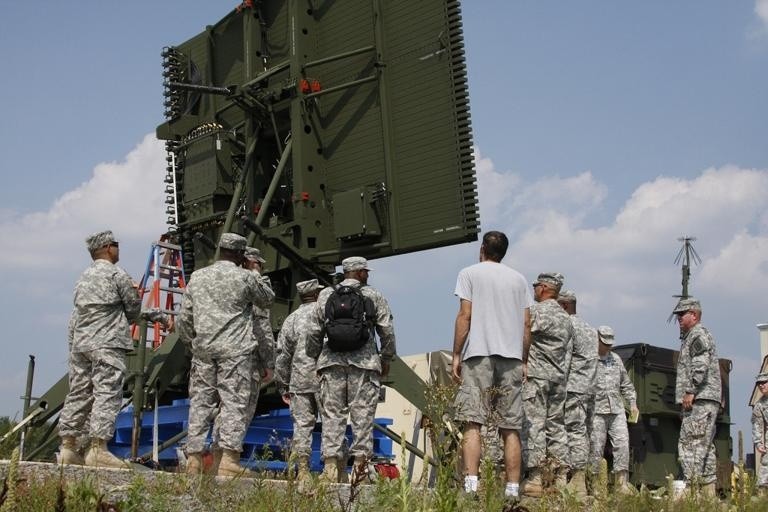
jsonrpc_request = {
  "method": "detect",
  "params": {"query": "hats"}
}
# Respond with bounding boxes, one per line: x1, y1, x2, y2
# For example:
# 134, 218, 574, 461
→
598, 326, 615, 345
756, 374, 768, 382
673, 299, 703, 312
296, 279, 325, 295
86, 230, 119, 253
220, 232, 265, 262
342, 257, 373, 273
534, 272, 578, 301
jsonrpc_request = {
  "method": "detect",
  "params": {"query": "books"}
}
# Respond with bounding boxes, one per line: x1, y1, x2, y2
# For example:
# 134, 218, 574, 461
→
628, 409, 641, 425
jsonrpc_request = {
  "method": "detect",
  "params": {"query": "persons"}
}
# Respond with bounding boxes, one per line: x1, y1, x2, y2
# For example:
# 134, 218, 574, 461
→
142, 305, 176, 335
751, 373, 768, 502
274, 267, 348, 483
304, 255, 398, 484
589, 326, 641, 501
672, 296, 724, 503
520, 270, 575, 499
214, 244, 276, 476
451, 229, 534, 503
558, 291, 601, 506
175, 232, 276, 479
57, 229, 142, 470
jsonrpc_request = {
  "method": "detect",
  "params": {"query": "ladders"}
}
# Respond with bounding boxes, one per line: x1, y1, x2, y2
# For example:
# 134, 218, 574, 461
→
129, 239, 188, 352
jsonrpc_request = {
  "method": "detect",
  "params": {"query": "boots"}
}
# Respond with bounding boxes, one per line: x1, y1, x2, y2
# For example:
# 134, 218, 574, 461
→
521, 465, 719, 499
217, 450, 261, 477
85, 442, 128, 468
295, 456, 370, 483
59, 444, 83, 464
185, 452, 205, 475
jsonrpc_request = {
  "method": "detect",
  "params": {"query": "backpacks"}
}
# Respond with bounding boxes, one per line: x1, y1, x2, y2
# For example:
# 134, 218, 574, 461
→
325, 284, 374, 352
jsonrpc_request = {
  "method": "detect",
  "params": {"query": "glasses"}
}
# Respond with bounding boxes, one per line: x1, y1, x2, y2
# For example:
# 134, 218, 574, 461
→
103, 243, 118, 248
